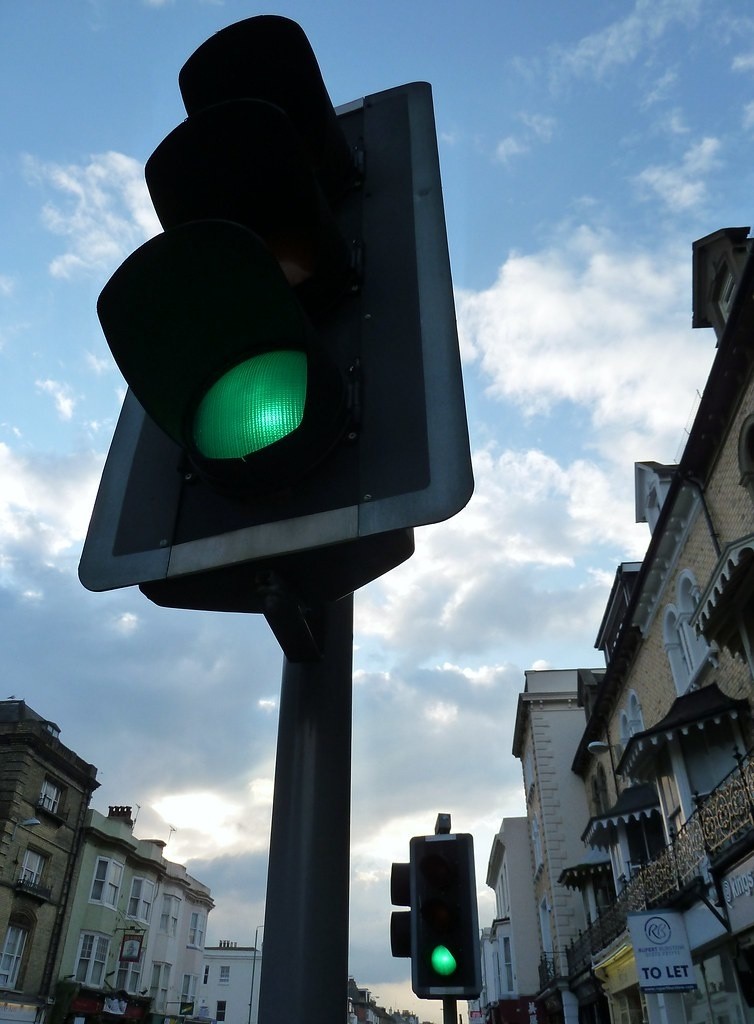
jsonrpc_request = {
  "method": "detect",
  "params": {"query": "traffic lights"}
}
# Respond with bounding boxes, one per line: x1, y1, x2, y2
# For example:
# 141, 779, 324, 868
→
96, 16, 360, 503
411, 830, 484, 1001
390, 862, 412, 958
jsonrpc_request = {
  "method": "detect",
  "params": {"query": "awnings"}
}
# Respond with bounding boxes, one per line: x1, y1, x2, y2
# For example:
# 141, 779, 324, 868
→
556, 532, 754, 891
